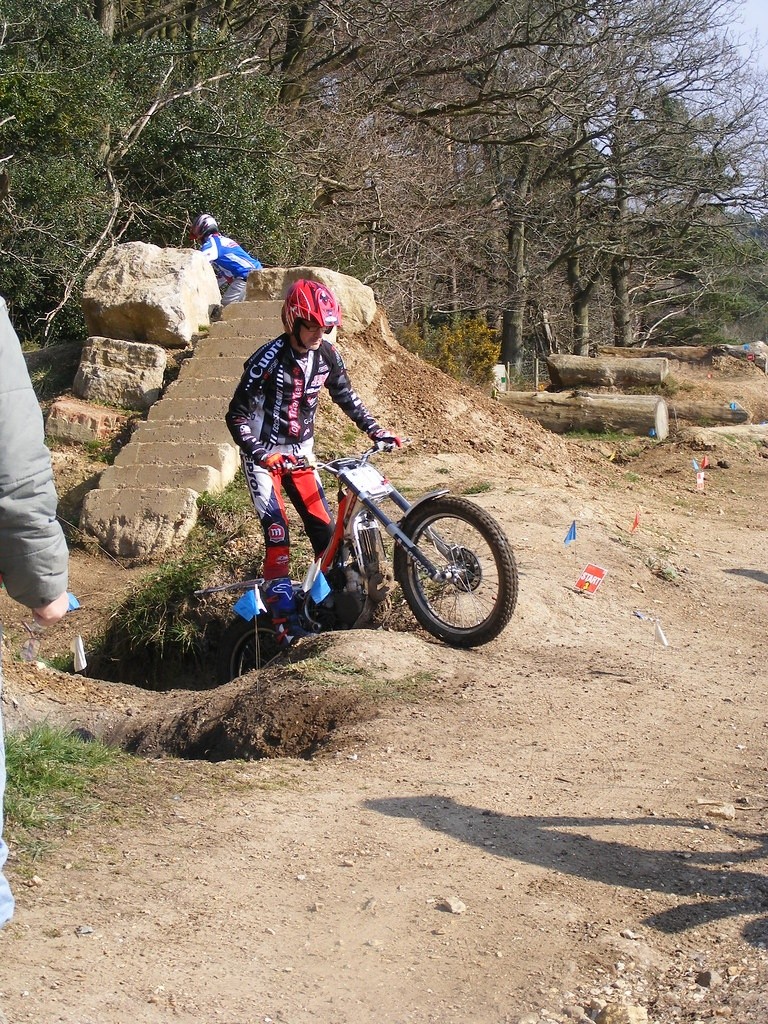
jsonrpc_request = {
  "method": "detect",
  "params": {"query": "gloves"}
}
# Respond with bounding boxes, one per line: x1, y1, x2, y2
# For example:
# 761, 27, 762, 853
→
371, 430, 401, 452
258, 453, 295, 477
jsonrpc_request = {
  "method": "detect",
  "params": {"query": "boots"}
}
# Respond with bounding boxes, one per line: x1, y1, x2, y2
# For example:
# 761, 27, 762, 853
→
264, 576, 321, 657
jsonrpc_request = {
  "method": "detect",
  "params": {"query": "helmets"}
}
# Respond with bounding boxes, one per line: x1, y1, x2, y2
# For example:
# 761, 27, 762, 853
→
190, 214, 219, 240
281, 279, 341, 335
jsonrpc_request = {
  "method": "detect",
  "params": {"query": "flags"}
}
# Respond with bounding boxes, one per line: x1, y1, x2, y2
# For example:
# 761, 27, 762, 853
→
631, 515, 638, 532
692, 454, 707, 473
69, 636, 87, 672
563, 521, 575, 545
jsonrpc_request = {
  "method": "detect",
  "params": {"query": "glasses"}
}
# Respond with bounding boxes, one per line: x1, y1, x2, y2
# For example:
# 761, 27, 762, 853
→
301, 322, 330, 332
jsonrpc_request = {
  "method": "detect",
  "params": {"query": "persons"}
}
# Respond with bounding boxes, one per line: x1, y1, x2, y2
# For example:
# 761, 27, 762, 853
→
0, 292, 71, 627
226, 278, 400, 644
188, 213, 261, 304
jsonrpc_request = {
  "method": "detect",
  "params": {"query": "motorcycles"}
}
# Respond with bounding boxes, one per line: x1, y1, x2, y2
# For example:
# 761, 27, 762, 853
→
193, 439, 520, 684
